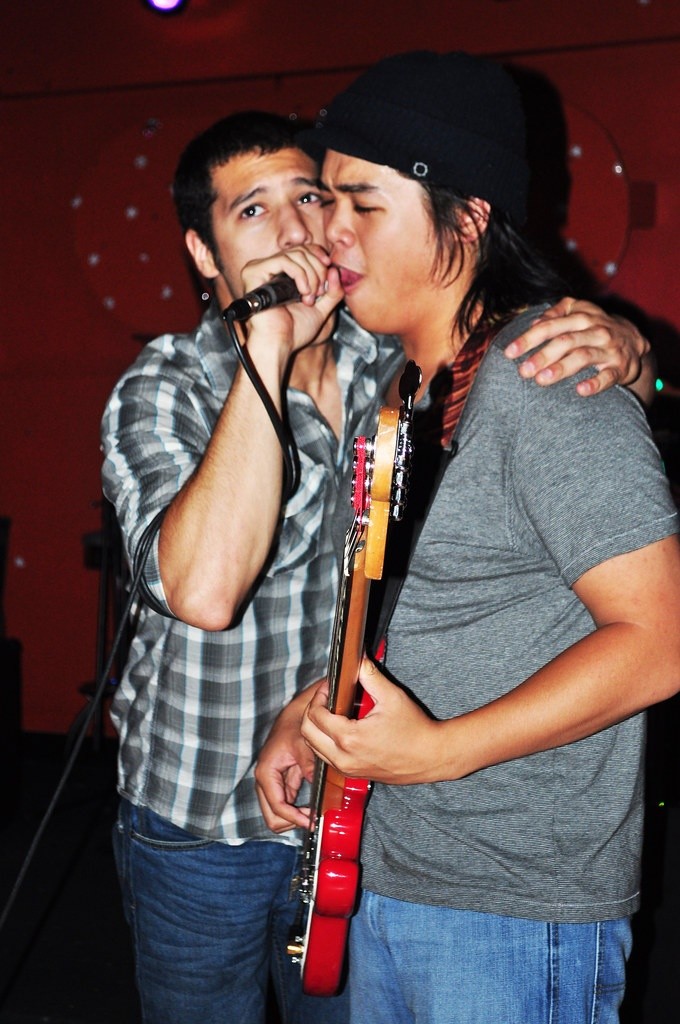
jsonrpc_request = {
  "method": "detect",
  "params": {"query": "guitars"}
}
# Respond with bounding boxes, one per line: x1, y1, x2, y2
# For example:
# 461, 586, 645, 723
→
287, 359, 423, 998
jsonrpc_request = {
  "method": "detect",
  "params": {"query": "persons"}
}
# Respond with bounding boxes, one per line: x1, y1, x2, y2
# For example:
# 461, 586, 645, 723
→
99, 111, 666, 1024
273, 48, 680, 1024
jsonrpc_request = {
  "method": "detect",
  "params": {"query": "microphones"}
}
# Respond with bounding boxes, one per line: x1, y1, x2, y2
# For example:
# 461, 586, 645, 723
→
220, 271, 303, 323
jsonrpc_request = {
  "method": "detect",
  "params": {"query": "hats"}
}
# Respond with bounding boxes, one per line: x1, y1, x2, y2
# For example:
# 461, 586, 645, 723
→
316, 52, 527, 225
